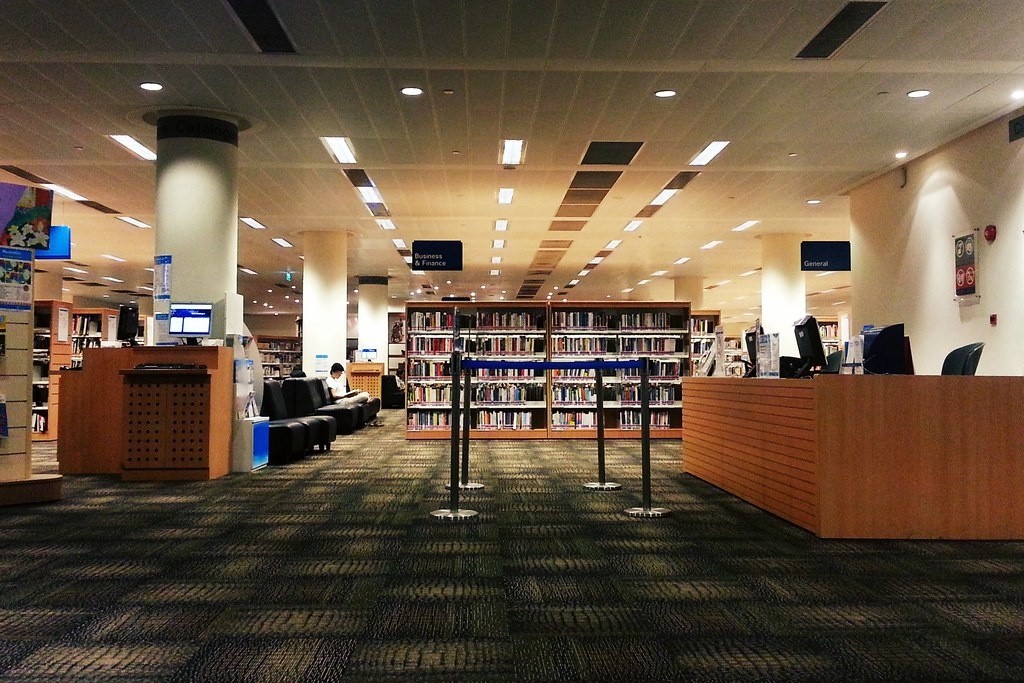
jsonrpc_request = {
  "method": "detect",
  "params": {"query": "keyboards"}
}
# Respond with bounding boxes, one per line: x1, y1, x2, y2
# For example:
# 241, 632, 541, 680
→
131, 361, 196, 369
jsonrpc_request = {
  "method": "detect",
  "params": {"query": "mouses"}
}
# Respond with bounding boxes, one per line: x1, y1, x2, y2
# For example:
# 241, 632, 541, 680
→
198, 364, 207, 370
59, 366, 70, 370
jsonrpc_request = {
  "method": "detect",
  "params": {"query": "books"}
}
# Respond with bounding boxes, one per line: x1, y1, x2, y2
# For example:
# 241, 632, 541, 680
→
409, 410, 533, 431
691, 318, 714, 376
552, 311, 672, 331
408, 384, 547, 404
818, 324, 839, 356
409, 310, 537, 330
552, 383, 677, 404
70, 316, 99, 368
551, 336, 676, 356
551, 359, 680, 380
32, 328, 50, 431
551, 410, 671, 430
409, 358, 546, 380
258, 343, 301, 375
409, 335, 548, 356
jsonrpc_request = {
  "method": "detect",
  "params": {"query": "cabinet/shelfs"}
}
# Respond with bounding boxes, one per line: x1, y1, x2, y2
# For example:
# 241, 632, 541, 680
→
251, 334, 303, 381
404, 299, 750, 441
29, 297, 154, 442
813, 315, 840, 365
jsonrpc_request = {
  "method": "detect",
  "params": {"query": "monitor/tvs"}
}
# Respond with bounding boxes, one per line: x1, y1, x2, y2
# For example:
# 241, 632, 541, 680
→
167, 301, 212, 347
115, 303, 142, 347
744, 324, 763, 378
793, 317, 829, 380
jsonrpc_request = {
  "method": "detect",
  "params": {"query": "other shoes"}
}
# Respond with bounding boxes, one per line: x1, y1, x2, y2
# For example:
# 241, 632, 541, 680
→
367, 420, 385, 427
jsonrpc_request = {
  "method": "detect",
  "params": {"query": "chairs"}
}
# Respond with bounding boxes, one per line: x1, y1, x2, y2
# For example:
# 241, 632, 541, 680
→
258, 376, 406, 459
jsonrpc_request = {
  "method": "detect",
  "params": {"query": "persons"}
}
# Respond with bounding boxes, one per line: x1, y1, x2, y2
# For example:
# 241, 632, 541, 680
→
290, 370, 307, 377
326, 363, 369, 403
395, 368, 404, 388
